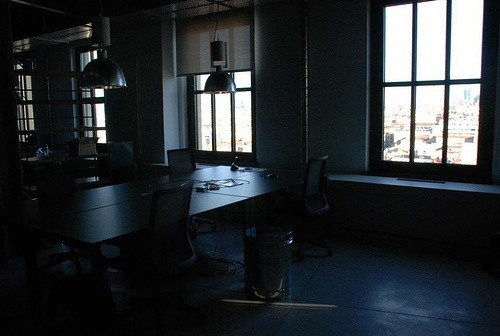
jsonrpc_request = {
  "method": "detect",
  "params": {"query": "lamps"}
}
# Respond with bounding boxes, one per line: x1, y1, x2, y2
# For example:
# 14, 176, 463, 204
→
204, 0, 238, 94
76, 0, 129, 90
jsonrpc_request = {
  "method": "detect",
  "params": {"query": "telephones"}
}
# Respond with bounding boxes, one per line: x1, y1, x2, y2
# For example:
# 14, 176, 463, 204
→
231, 156, 239, 171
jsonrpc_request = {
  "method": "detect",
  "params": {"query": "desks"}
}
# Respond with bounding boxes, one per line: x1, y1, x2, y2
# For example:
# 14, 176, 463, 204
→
0, 163, 304, 336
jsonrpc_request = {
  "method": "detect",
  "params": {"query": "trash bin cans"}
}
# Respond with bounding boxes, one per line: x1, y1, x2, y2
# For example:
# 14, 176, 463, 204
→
242, 225, 293, 305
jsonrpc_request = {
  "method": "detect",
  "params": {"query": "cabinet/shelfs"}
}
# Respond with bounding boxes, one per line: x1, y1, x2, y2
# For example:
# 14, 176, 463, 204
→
14, 68, 111, 145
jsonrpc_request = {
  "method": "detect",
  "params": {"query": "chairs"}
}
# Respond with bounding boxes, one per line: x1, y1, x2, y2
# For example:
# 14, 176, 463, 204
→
167, 148, 196, 176
26, 162, 58, 192
118, 184, 208, 336
266, 155, 335, 262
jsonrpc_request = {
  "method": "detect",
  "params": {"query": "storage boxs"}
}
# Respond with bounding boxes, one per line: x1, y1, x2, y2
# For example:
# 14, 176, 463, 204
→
64, 141, 97, 156
